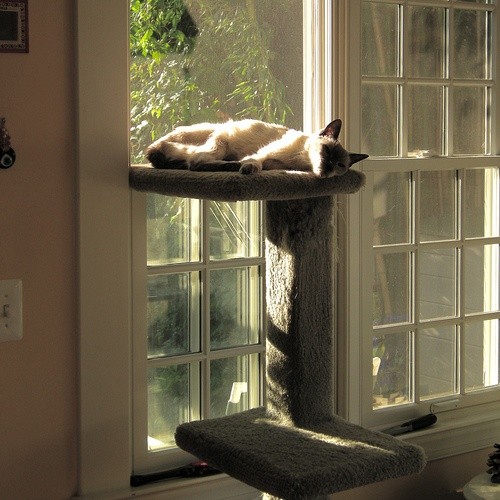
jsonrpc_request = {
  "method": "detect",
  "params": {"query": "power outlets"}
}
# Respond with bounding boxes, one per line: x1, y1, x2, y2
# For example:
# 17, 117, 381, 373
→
0, 277, 23, 341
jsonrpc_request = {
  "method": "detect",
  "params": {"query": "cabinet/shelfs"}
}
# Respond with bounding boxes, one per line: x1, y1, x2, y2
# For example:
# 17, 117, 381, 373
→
129, 161, 430, 499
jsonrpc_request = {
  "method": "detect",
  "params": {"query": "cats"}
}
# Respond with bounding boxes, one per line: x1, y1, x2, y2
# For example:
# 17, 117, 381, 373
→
145, 119, 368, 176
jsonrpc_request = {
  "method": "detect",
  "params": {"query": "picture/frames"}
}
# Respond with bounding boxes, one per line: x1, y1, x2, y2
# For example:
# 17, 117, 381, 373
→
0, 0, 30, 54
